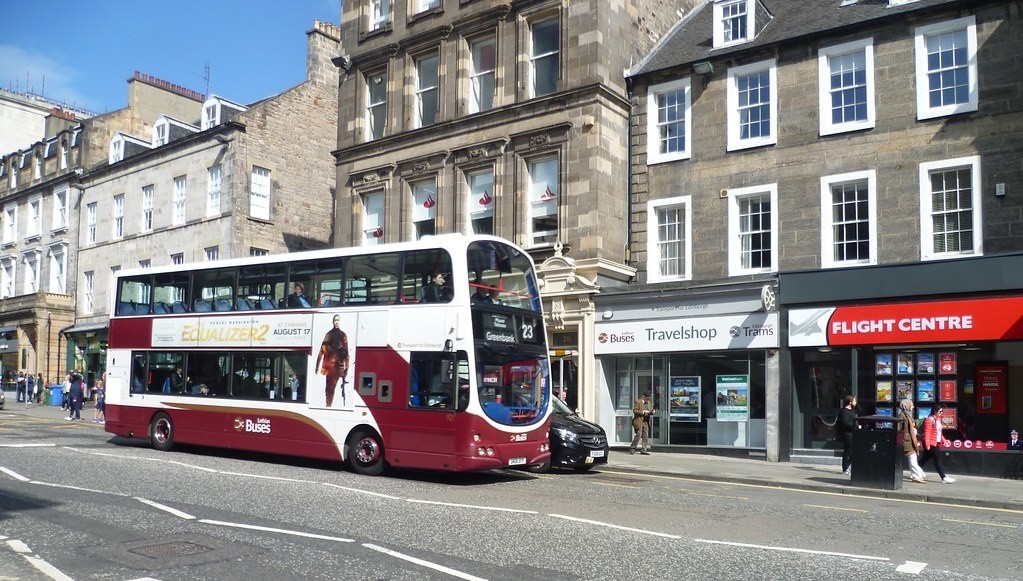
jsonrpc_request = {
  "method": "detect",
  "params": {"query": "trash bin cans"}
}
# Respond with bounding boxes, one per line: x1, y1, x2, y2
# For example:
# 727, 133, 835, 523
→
45, 385, 63, 406
850, 416, 906, 490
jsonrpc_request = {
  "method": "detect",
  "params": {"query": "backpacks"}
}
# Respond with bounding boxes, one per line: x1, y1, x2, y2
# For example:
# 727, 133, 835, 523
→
917, 417, 934, 441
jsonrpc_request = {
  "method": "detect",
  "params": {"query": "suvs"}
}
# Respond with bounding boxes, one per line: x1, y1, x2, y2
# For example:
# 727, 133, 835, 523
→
460, 381, 609, 472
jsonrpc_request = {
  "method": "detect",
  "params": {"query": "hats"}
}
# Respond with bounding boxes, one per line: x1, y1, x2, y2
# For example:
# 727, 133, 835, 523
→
643, 392, 651, 398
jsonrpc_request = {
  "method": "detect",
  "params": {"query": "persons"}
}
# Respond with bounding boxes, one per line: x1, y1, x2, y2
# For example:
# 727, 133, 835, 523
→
1008, 428, 1023, 450
557, 390, 567, 406
60, 369, 106, 423
288, 283, 312, 307
1, 369, 44, 405
838, 393, 857, 475
918, 403, 956, 484
628, 392, 655, 454
315, 314, 350, 407
170, 358, 299, 400
896, 399, 927, 483
423, 268, 504, 304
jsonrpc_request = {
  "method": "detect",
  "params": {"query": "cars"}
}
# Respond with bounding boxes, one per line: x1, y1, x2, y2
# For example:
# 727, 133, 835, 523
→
0, 388, 5, 410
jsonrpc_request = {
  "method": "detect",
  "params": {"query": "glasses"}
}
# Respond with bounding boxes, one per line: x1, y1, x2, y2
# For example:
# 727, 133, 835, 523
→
940, 411, 943, 413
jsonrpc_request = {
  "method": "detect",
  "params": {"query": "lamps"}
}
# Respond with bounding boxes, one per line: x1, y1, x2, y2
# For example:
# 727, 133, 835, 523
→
213, 134, 229, 147
693, 62, 714, 90
71, 183, 86, 194
331, 55, 352, 74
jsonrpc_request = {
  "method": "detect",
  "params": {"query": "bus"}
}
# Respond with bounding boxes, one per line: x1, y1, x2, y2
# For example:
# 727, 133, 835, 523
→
105, 232, 551, 476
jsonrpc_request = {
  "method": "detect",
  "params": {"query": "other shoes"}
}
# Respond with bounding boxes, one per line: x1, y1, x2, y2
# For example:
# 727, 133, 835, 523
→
67, 408, 69, 411
61, 407, 64, 410
629, 448, 635, 454
843, 467, 851, 476
942, 474, 955, 483
65, 416, 72, 420
90, 417, 105, 423
641, 452, 649, 455
912, 479, 926, 483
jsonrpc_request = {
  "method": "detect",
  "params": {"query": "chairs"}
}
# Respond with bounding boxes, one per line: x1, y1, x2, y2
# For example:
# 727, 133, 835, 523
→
119, 293, 345, 315
135, 375, 201, 394
